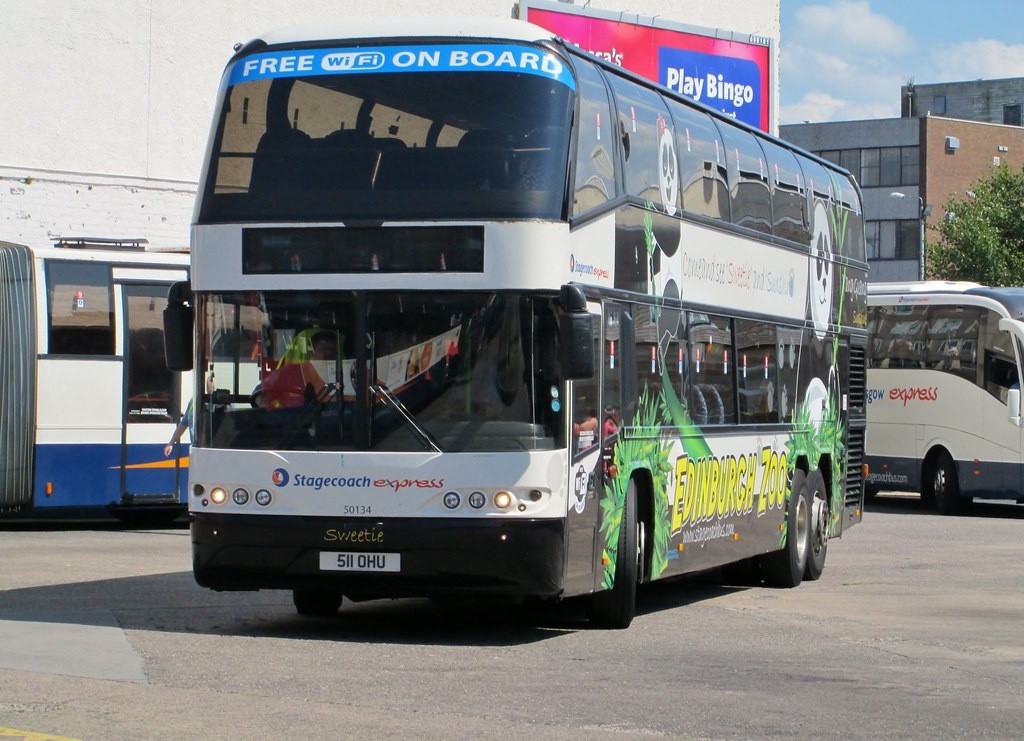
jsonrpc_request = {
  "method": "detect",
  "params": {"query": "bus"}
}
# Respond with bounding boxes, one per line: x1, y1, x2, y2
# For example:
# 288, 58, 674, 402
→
160, 32, 868, 630
0, 166, 298, 533
866, 281, 1024, 515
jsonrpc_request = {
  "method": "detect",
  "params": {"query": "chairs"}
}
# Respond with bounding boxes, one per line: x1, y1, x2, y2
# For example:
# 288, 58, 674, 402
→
692, 383, 736, 424
249, 127, 564, 195
49, 325, 258, 400
